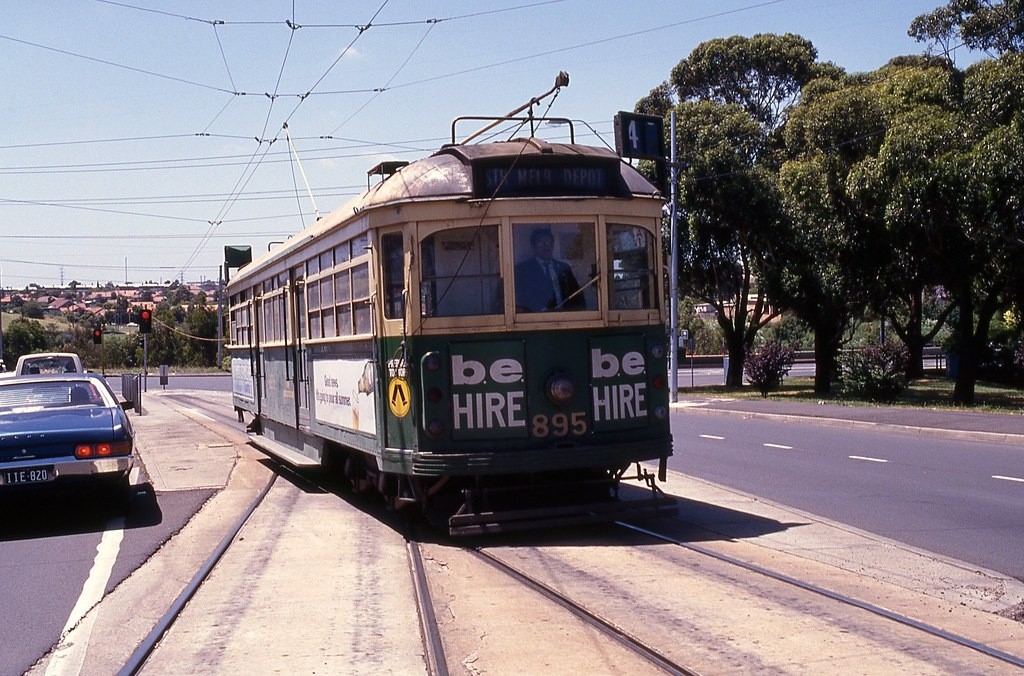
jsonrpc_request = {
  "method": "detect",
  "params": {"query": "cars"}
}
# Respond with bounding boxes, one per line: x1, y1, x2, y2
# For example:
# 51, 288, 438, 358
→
14, 351, 85, 376
0, 372, 135, 489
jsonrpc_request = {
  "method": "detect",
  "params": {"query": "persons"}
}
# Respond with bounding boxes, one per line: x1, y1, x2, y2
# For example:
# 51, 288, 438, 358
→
0, 358, 6, 373
514, 228, 590, 312
65, 362, 74, 371
71, 388, 90, 403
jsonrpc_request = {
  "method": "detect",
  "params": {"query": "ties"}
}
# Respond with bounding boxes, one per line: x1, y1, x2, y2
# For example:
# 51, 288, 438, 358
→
542, 261, 553, 285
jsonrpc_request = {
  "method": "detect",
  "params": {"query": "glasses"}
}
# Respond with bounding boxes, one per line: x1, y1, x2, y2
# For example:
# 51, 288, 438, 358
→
533, 239, 554, 247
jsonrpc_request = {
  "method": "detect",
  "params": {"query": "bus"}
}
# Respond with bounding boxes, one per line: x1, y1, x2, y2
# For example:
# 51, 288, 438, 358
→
227, 137, 678, 535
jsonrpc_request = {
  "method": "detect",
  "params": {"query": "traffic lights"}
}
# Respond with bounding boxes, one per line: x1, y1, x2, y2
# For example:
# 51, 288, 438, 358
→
93, 328, 102, 344
139, 309, 152, 333
